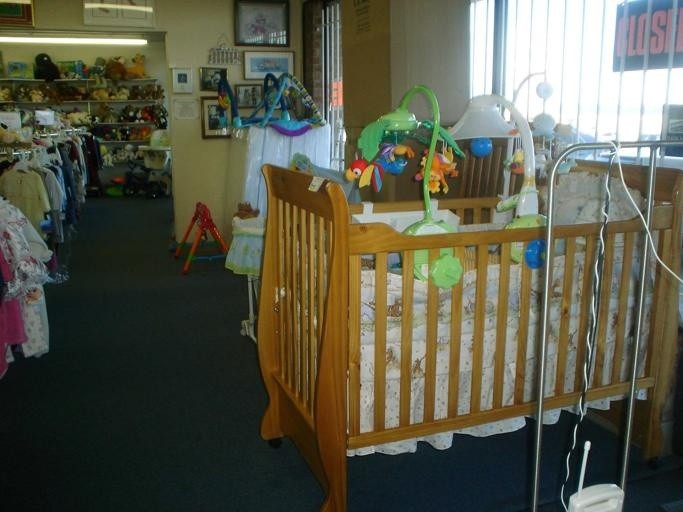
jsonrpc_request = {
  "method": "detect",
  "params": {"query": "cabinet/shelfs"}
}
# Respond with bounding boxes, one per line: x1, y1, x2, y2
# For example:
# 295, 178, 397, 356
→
0, 77, 163, 148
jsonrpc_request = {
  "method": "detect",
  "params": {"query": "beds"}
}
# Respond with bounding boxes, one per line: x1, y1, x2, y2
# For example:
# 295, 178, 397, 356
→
256, 159, 682, 512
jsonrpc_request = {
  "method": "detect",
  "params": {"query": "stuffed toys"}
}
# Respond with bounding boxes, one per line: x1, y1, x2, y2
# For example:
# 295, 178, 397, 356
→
0, 52, 172, 200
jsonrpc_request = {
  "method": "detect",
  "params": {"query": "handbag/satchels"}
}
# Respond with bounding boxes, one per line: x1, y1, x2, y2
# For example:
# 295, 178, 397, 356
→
36, 54, 59, 78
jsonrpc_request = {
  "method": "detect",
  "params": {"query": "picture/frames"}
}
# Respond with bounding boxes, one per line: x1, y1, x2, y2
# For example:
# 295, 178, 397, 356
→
80, 0, 158, 30
0, 0, 37, 30
170, 1, 295, 140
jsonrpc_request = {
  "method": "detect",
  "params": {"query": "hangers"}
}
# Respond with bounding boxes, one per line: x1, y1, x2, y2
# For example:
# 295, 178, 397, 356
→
0, 120, 95, 175
